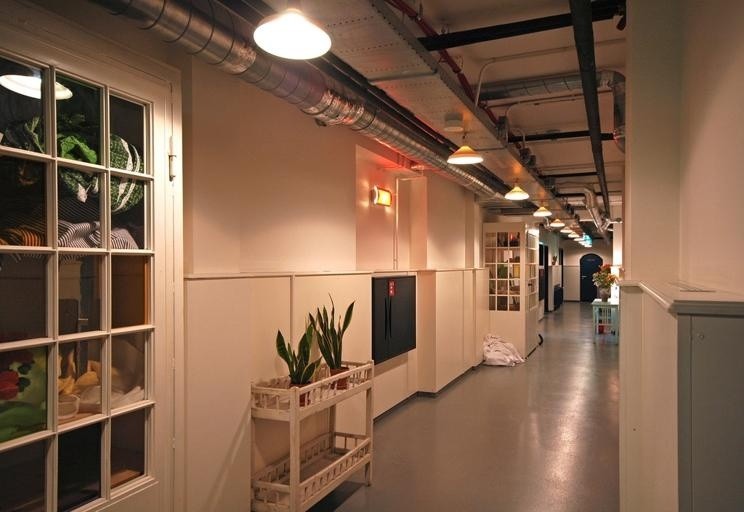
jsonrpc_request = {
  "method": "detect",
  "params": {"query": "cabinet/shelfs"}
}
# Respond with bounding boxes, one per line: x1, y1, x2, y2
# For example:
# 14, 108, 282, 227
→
483, 221, 536, 362
1, 0, 187, 512
250, 360, 374, 511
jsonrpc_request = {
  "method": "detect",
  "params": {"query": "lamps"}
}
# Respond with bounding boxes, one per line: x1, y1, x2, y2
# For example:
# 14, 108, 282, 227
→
447, 130, 551, 217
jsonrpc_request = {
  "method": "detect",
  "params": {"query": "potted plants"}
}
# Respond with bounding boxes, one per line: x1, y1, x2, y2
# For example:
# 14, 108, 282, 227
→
276, 293, 355, 407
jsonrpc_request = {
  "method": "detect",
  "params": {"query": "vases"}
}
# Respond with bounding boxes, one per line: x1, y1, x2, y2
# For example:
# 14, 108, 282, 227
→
598, 286, 610, 302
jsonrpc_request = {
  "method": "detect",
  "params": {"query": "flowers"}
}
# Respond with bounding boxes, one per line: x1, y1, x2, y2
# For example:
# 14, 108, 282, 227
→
592, 262, 620, 287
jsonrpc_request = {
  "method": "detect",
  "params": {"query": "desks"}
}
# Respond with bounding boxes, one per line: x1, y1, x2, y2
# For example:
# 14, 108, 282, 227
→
591, 297, 618, 337
